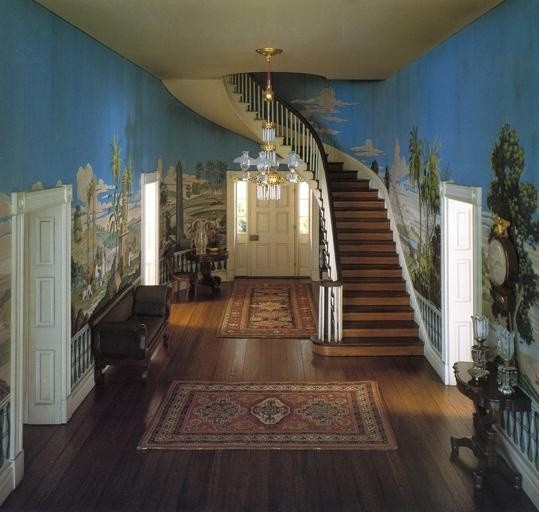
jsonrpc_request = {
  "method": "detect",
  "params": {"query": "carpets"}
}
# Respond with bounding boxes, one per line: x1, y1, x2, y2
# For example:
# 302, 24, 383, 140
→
217, 278, 318, 338
137, 380, 399, 451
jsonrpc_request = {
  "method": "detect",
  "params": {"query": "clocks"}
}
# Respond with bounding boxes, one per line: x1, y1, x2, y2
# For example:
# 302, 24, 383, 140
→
486, 216, 518, 312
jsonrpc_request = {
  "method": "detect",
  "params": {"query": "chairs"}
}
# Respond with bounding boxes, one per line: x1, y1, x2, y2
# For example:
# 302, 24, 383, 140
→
166, 248, 194, 303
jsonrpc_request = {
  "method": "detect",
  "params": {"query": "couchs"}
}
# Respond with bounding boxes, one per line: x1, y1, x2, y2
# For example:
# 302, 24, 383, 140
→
88, 283, 172, 386
232, 47, 304, 201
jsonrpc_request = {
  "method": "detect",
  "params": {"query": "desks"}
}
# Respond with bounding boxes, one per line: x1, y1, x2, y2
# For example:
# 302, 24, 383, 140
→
449, 362, 531, 491
185, 252, 229, 298
